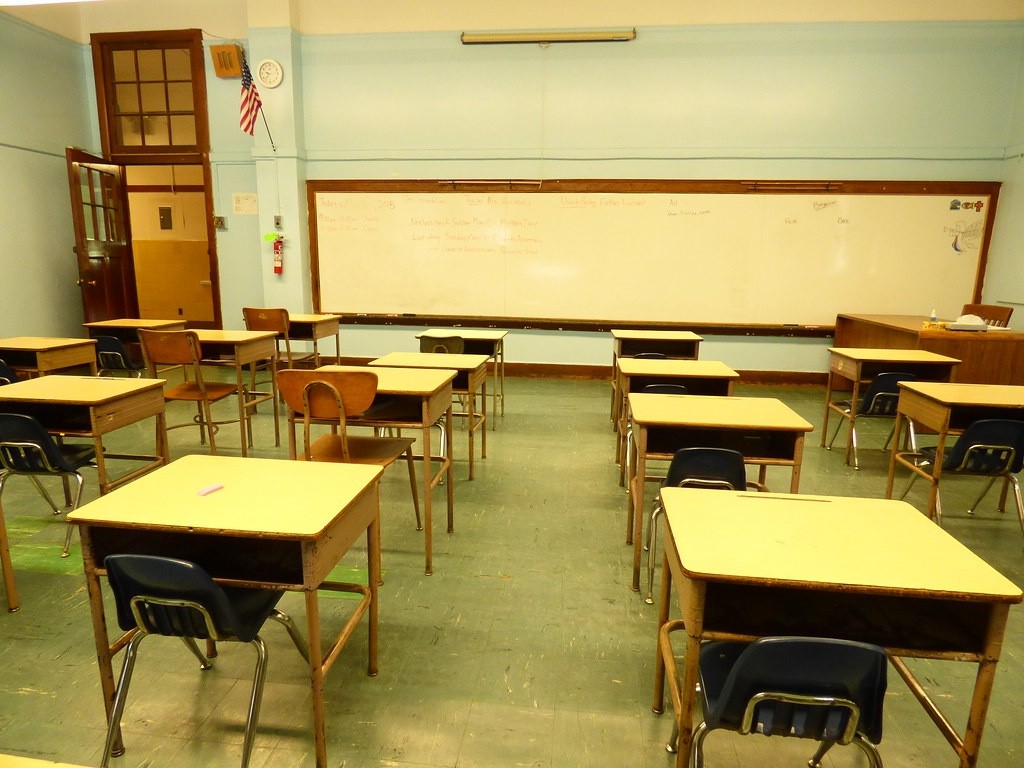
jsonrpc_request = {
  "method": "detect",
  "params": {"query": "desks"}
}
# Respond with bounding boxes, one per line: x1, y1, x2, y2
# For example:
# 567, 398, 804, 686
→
0, 313, 1024, 768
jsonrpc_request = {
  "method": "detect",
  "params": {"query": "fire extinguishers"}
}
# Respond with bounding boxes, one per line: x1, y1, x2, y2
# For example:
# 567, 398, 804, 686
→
273, 241, 283, 273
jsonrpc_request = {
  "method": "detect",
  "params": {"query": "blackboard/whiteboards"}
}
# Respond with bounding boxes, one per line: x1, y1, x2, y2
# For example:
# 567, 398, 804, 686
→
306, 178, 1003, 339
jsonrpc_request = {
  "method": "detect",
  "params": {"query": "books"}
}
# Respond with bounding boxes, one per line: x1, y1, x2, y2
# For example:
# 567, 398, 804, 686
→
347, 397, 400, 419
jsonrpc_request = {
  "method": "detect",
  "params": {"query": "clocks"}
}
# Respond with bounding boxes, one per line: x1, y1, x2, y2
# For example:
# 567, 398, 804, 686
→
256, 58, 283, 89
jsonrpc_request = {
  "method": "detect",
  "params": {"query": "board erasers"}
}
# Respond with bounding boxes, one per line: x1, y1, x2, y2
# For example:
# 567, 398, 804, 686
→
783, 323, 799, 327
402, 314, 416, 318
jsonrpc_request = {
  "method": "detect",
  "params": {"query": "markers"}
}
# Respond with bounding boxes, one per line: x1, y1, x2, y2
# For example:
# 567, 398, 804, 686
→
803, 325, 820, 328
356, 313, 367, 316
324, 314, 333, 315
384, 314, 397, 317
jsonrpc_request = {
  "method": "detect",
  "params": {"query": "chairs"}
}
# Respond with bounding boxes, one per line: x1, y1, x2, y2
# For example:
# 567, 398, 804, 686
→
625, 384, 689, 493
642, 447, 746, 606
633, 353, 666, 359
959, 304, 1013, 327
97, 554, 307, 768
419, 335, 477, 431
92, 335, 144, 379
897, 416, 1024, 533
274, 367, 423, 588
663, 634, 888, 768
0, 412, 119, 558
826, 370, 919, 471
0, 359, 20, 387
242, 307, 322, 413
136, 327, 253, 457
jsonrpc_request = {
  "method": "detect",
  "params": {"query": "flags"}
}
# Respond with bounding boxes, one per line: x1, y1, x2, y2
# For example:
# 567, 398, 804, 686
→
238, 51, 261, 138
